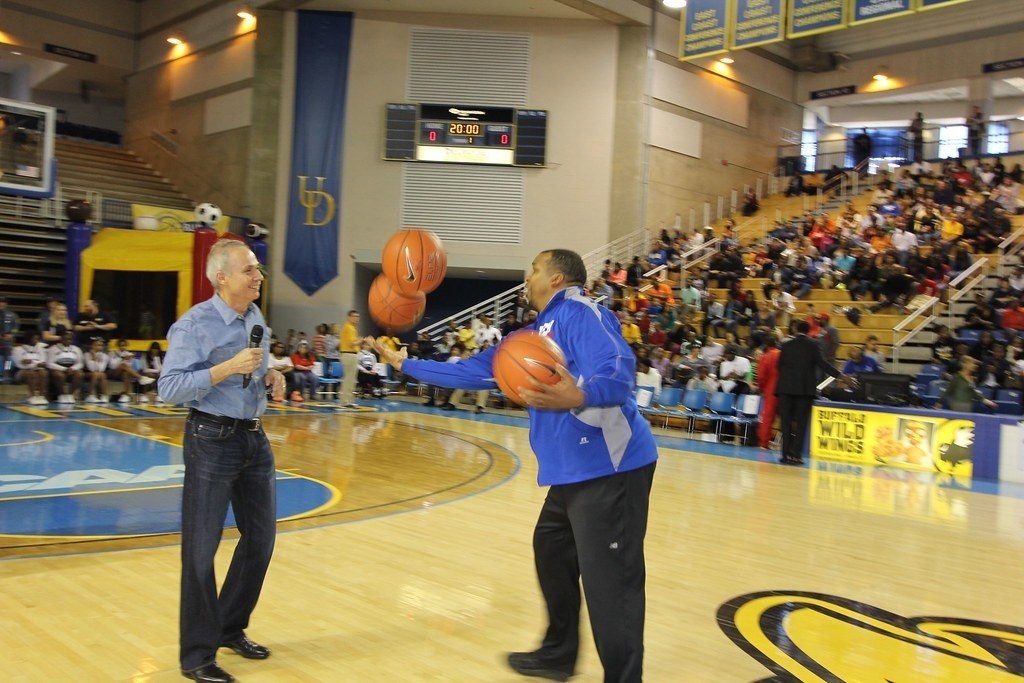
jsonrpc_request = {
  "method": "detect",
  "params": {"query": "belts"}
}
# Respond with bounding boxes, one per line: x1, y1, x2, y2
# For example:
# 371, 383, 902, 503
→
191, 408, 262, 431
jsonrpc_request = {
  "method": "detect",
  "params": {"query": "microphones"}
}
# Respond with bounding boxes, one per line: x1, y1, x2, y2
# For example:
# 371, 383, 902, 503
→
242, 324, 264, 389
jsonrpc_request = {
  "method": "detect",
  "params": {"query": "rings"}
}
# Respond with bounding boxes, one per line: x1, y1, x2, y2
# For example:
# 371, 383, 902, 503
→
282, 385, 286, 388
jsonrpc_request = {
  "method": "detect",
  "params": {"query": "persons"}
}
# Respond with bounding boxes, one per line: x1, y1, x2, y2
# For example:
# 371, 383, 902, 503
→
773, 322, 860, 465
373, 250, 659, 683
0, 299, 400, 408
0, 113, 32, 154
404, 109, 1024, 453
157, 240, 286, 683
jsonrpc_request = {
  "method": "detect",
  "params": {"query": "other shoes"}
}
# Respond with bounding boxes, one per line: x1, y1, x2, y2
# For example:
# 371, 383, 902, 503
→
509, 652, 568, 682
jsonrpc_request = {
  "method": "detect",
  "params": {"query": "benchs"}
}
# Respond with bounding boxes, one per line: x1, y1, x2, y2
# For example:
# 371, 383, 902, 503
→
637, 188, 1023, 376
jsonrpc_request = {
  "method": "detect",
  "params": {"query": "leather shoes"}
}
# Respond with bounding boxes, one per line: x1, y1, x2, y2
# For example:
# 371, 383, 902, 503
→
219, 635, 269, 659
182, 663, 234, 683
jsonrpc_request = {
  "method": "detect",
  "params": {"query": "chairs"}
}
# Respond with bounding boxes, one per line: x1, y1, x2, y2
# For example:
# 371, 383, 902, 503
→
635, 385, 762, 447
911, 330, 1024, 417
128, 358, 141, 405
0, 355, 16, 385
309, 361, 507, 410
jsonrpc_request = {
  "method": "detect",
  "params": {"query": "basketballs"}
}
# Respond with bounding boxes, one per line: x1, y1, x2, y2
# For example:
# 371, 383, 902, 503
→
381, 227, 448, 297
491, 328, 568, 408
367, 272, 426, 327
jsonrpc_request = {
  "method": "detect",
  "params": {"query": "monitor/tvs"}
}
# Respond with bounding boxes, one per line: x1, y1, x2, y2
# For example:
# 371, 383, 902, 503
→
853, 372, 909, 406
